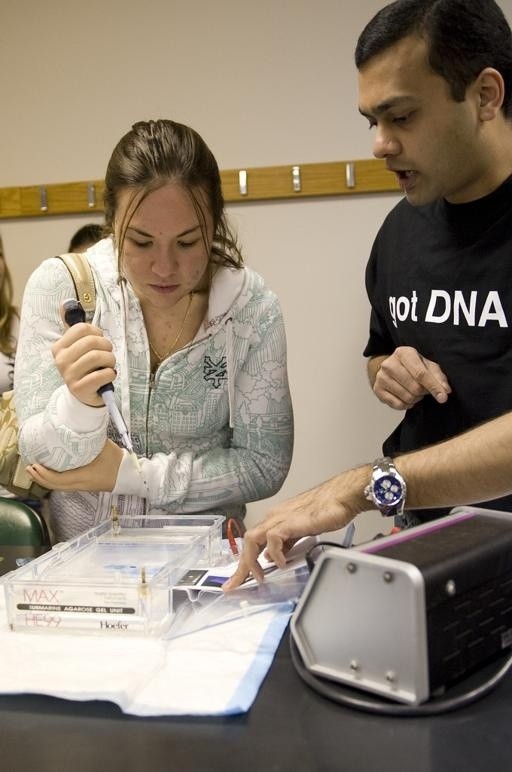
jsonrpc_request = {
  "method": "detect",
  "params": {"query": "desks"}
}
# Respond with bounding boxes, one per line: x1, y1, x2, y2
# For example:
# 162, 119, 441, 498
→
0, 534, 511, 767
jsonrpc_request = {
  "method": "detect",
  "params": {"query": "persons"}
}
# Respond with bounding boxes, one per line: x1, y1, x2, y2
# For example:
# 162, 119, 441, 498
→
13, 117, 296, 538
67, 221, 105, 253
0, 236, 17, 497
217, 0, 512, 600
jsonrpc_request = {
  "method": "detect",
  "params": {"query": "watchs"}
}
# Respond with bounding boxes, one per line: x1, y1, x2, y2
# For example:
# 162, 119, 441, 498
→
363, 455, 406, 520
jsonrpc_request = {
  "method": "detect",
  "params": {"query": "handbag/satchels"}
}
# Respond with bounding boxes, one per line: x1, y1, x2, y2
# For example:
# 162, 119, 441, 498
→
0, 385, 56, 503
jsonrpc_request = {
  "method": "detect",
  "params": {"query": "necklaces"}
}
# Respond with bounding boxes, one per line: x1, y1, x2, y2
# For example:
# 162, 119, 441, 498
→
150, 291, 195, 372
